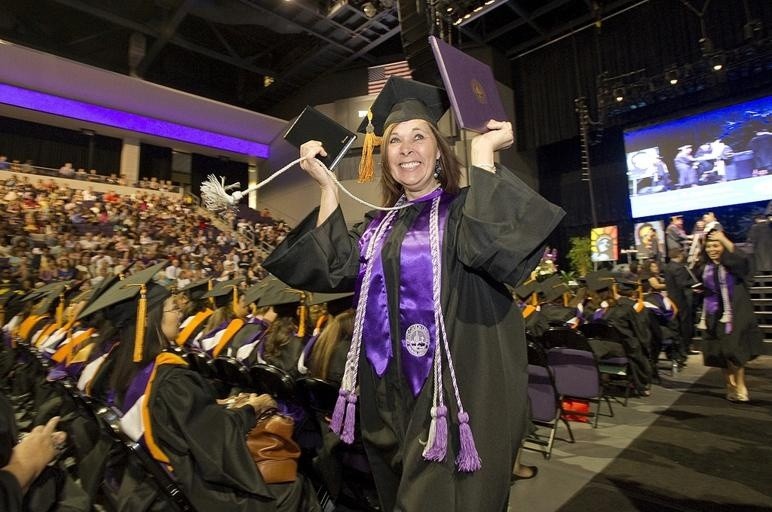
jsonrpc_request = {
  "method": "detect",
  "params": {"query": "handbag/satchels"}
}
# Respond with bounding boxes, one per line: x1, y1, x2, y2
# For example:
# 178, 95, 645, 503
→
247, 413, 301, 483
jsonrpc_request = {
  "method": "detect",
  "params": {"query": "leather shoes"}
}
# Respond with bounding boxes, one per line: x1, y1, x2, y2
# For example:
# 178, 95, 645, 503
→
512, 466, 537, 480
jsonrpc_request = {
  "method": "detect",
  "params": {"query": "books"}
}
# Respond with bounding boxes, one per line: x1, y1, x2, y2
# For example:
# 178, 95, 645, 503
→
425, 35, 510, 138
282, 104, 358, 173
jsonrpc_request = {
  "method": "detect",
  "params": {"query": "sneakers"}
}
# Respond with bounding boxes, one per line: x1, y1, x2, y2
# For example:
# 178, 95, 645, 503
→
727, 383, 749, 401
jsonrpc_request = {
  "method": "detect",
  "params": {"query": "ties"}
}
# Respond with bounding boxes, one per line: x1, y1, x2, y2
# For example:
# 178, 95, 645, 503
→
381, 205, 421, 380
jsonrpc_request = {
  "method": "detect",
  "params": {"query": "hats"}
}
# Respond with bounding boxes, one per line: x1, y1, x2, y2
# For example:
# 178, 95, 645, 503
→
669, 215, 683, 220
357, 76, 451, 183
516, 273, 651, 307
678, 145, 692, 151
706, 228, 725, 241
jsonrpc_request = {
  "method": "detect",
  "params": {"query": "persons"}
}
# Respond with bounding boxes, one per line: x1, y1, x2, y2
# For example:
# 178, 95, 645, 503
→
0, 156, 355, 512
649, 125, 772, 195
511, 203, 772, 485
260, 113, 569, 512
